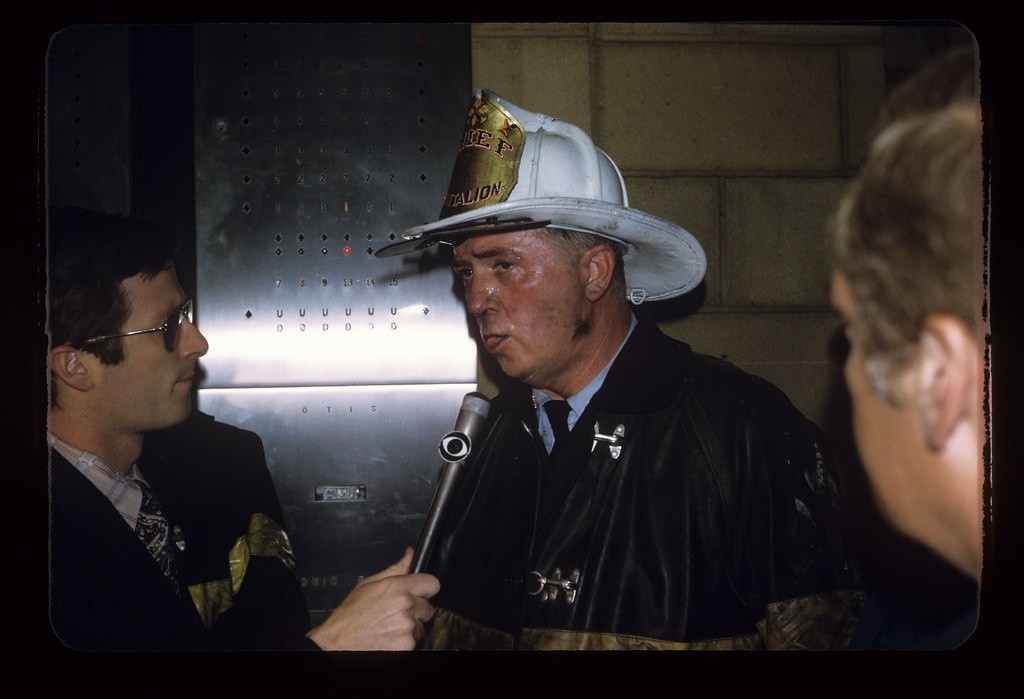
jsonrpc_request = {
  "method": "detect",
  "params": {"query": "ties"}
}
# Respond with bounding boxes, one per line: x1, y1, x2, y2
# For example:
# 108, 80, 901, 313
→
134, 483, 186, 578
543, 399, 572, 538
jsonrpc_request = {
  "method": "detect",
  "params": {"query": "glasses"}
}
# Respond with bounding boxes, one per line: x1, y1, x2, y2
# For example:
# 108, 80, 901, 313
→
84, 300, 193, 353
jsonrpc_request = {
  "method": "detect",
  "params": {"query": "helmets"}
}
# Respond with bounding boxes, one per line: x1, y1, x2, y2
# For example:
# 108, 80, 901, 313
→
373, 88, 707, 299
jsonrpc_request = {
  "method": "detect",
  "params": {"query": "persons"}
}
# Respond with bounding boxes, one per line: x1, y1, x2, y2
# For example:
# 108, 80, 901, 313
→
828, 43, 992, 584
47, 206, 442, 651
375, 87, 869, 651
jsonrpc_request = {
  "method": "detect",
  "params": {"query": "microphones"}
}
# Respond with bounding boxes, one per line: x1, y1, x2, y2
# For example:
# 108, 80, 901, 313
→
408, 391, 491, 576
